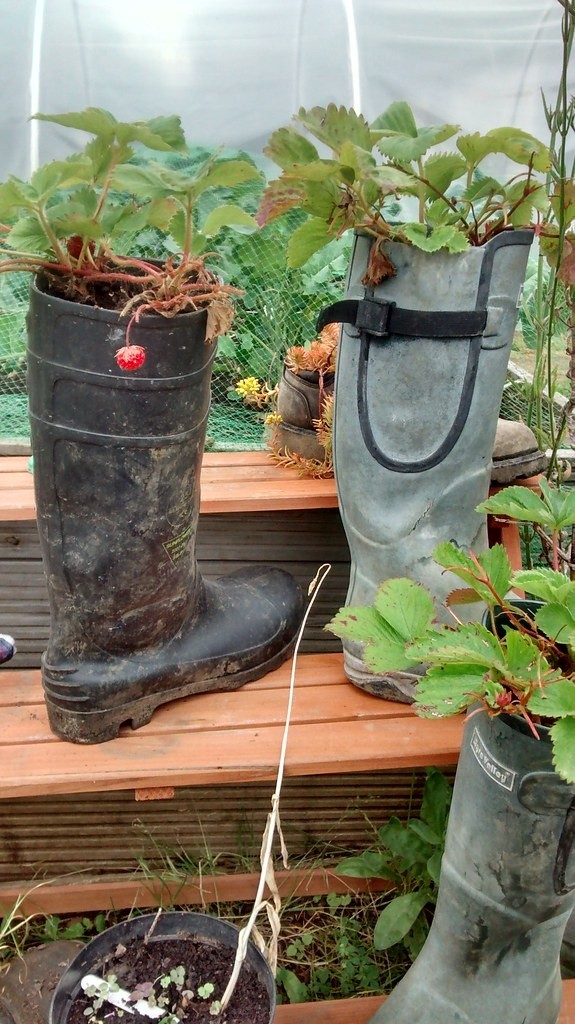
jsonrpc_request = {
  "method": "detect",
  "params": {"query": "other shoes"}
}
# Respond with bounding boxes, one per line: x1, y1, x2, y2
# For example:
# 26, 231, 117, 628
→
268, 358, 547, 481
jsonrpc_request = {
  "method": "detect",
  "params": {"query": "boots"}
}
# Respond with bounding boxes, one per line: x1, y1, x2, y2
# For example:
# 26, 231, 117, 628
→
312, 226, 534, 702
24, 254, 304, 743
366, 698, 575, 1023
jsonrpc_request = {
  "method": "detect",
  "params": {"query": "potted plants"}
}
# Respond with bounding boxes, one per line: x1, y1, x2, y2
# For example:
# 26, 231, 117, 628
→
49, 912, 277, 1024
